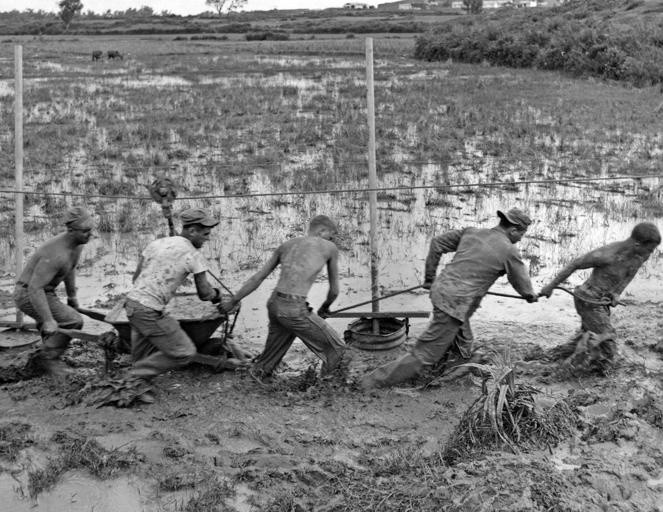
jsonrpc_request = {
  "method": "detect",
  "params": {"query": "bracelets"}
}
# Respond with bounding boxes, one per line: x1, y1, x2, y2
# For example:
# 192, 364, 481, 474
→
66, 295, 76, 300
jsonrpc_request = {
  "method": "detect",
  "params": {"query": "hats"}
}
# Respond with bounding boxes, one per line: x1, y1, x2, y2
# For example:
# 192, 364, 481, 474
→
495, 204, 533, 230
179, 208, 222, 230
62, 208, 95, 230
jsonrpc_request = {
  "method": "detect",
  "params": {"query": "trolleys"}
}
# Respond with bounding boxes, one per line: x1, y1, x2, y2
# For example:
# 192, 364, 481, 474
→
0, 292, 252, 371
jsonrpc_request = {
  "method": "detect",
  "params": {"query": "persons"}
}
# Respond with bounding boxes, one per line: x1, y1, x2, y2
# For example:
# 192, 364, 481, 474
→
217, 214, 349, 388
536, 221, 662, 384
11, 204, 93, 377
357, 206, 539, 399
120, 206, 224, 405
